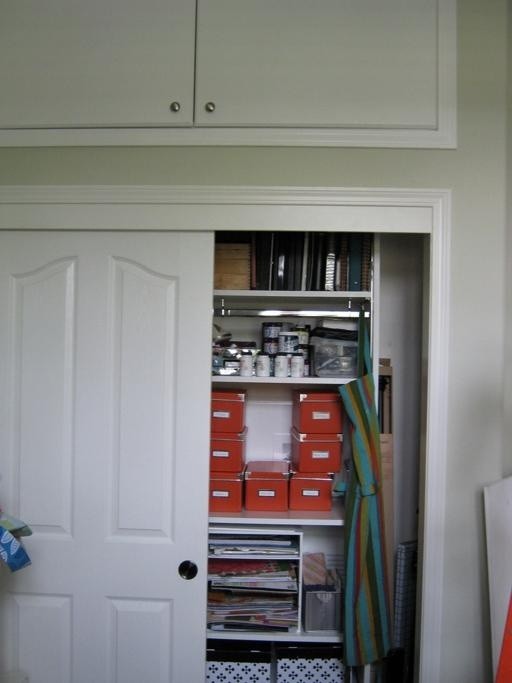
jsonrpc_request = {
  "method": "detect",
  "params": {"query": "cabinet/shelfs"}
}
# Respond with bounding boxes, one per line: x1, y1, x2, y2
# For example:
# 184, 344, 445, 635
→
207, 524, 303, 636
1, 184, 449, 682
0, 0, 458, 149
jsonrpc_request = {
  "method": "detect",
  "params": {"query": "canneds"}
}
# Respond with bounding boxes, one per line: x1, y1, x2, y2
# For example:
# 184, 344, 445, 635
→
262, 322, 310, 377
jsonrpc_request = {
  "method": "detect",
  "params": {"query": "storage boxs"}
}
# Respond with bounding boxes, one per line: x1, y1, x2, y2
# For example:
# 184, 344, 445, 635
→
308, 336, 359, 378
209, 390, 345, 514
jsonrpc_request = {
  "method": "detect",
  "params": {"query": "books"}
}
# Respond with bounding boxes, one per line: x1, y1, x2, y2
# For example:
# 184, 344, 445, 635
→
208, 537, 298, 628
251, 233, 375, 290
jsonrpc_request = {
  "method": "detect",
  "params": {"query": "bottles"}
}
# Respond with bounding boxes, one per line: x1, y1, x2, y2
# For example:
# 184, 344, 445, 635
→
238, 351, 305, 378
